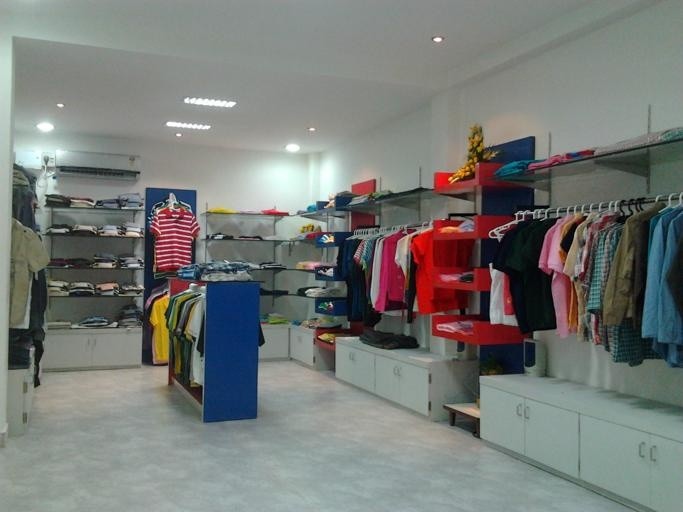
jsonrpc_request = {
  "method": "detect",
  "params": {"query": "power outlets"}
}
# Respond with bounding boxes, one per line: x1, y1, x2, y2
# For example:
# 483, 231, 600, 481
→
42, 152, 55, 168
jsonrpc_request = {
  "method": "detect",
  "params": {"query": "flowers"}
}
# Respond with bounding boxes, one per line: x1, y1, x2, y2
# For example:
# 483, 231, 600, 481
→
446, 123, 498, 184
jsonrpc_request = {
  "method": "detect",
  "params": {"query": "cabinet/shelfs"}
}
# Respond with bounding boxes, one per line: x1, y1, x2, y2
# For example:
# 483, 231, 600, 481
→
33, 202, 144, 373
334, 184, 477, 420
199, 206, 348, 368
468, 137, 683, 512
430, 163, 530, 349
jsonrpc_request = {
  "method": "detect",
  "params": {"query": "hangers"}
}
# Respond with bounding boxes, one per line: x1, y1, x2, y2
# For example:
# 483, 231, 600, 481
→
166, 192, 182, 213
486, 191, 683, 243
346, 221, 434, 241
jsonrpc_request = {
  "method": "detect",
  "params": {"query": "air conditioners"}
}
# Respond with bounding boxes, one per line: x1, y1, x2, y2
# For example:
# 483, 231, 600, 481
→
56, 149, 142, 181
